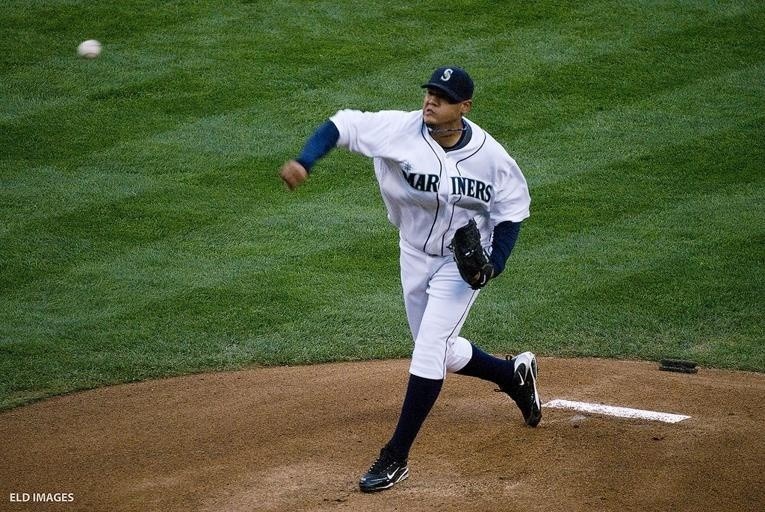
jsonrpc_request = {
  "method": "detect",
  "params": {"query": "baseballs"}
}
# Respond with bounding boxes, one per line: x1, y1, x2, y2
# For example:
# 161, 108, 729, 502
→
78, 39, 103, 58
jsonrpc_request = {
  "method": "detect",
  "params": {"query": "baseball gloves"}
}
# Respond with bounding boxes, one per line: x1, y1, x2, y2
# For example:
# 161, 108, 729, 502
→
446, 215, 493, 289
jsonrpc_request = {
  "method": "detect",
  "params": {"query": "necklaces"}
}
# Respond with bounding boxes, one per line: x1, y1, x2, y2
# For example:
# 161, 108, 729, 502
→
426, 120, 470, 133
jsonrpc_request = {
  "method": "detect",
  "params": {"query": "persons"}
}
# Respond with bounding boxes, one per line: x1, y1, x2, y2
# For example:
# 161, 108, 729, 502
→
277, 67, 541, 492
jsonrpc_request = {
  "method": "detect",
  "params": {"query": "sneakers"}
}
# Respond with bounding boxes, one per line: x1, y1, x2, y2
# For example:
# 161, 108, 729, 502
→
493, 350, 542, 428
359, 446, 409, 492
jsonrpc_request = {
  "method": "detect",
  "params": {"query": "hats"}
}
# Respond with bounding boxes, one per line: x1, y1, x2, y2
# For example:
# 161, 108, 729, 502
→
419, 64, 475, 104
